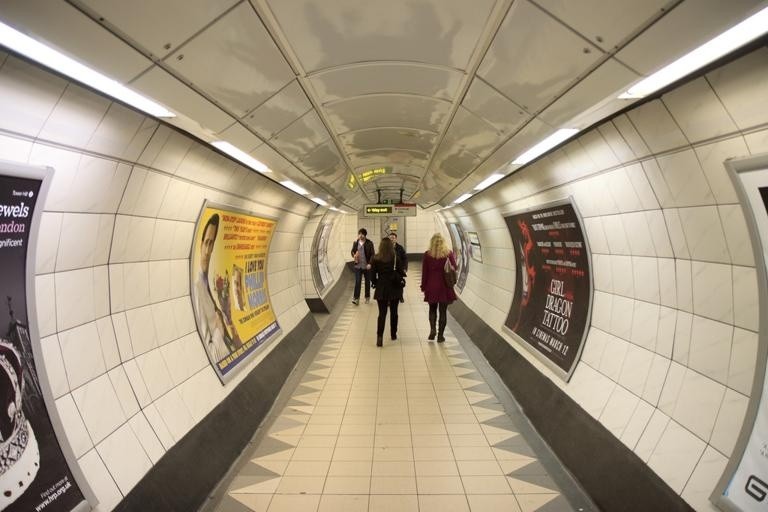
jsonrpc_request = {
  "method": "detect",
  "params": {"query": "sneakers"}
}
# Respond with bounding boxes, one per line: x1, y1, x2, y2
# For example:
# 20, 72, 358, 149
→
352, 298, 359, 305
365, 297, 369, 303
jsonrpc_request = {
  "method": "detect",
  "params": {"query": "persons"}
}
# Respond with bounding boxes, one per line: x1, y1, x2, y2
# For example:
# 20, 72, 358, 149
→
421, 232, 458, 342
235, 271, 245, 310
368, 237, 403, 347
505, 216, 549, 341
192, 213, 234, 365
387, 233, 407, 305
351, 229, 375, 305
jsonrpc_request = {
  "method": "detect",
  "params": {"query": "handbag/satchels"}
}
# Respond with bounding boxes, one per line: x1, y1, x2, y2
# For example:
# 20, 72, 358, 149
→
444, 258, 457, 289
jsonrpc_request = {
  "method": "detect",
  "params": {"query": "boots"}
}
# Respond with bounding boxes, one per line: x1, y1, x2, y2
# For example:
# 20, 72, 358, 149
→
437, 320, 447, 342
390, 314, 398, 340
428, 319, 436, 340
377, 317, 385, 347
